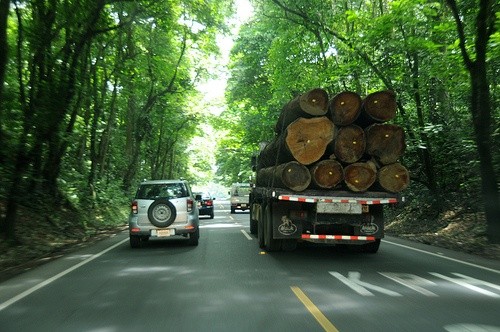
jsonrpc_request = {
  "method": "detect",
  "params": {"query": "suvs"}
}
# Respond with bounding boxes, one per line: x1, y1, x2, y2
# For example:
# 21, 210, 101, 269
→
129, 177, 199, 246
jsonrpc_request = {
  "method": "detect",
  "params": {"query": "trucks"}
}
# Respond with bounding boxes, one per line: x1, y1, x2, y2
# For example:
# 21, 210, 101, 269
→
228, 182, 256, 214
248, 140, 405, 255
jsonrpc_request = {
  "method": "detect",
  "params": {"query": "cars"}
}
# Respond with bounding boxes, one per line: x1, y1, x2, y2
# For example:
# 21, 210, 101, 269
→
191, 186, 216, 219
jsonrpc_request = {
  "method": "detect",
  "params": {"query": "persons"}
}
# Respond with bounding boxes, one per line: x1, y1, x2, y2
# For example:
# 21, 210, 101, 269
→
148, 188, 161, 199
